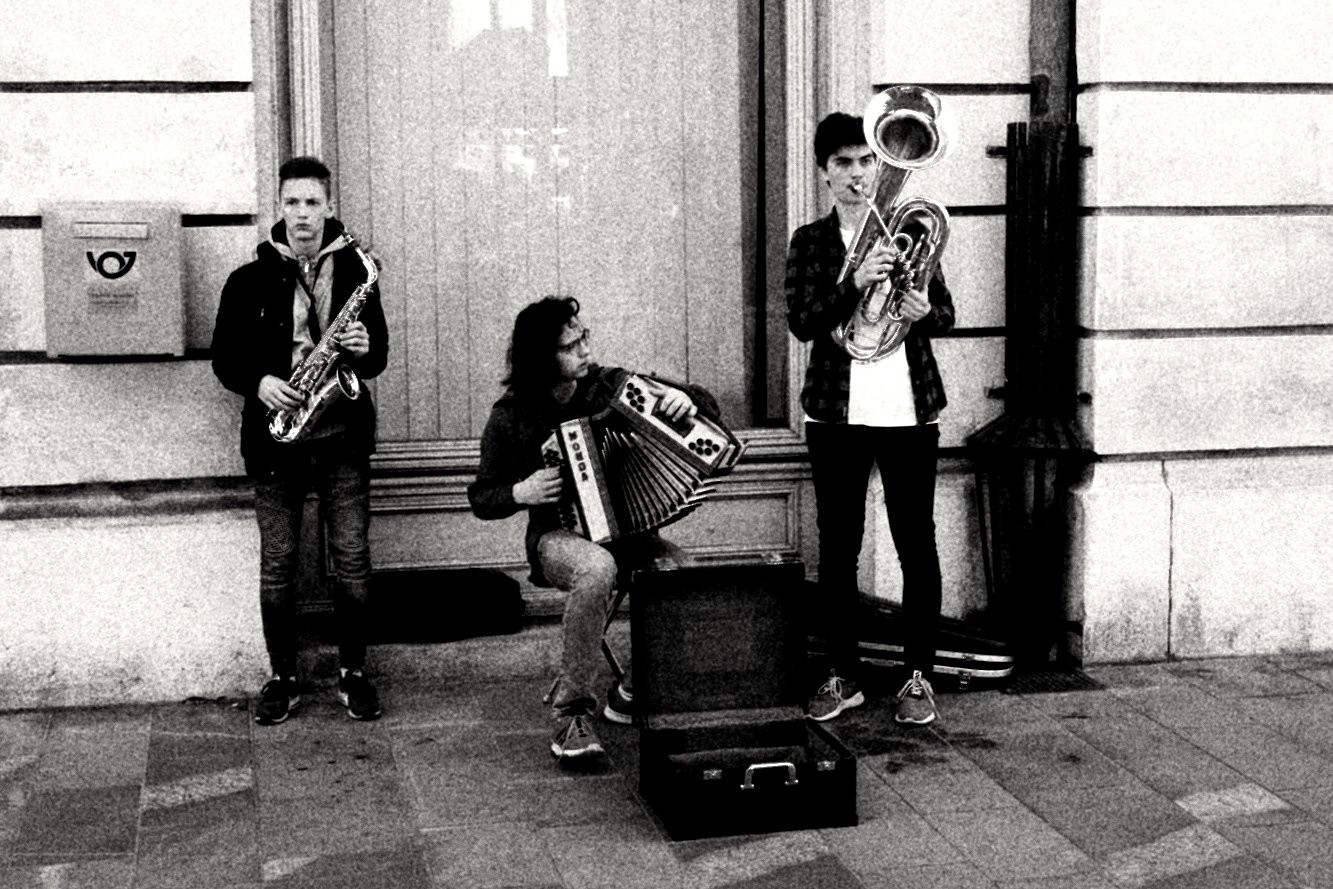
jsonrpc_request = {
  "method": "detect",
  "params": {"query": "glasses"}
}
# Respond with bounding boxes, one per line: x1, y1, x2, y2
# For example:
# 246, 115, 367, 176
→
556, 328, 591, 356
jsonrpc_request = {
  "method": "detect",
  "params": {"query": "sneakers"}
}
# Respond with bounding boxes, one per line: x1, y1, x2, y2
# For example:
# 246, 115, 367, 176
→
895, 670, 942, 724
256, 674, 300, 723
335, 668, 381, 720
604, 684, 634, 724
551, 718, 605, 758
805, 671, 866, 722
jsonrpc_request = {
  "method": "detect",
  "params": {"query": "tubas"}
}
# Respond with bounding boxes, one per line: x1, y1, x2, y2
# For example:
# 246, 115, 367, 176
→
829, 83, 960, 366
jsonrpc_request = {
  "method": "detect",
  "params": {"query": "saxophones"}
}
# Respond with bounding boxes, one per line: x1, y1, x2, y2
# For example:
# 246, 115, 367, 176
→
266, 225, 381, 445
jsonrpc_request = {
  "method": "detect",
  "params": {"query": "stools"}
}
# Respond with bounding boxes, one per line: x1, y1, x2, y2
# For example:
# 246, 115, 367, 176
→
525, 553, 626, 702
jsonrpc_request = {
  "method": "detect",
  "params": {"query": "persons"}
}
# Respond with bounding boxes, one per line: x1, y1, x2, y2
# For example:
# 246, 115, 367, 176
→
467, 295, 721, 757
785, 111, 955, 725
211, 157, 390, 723
